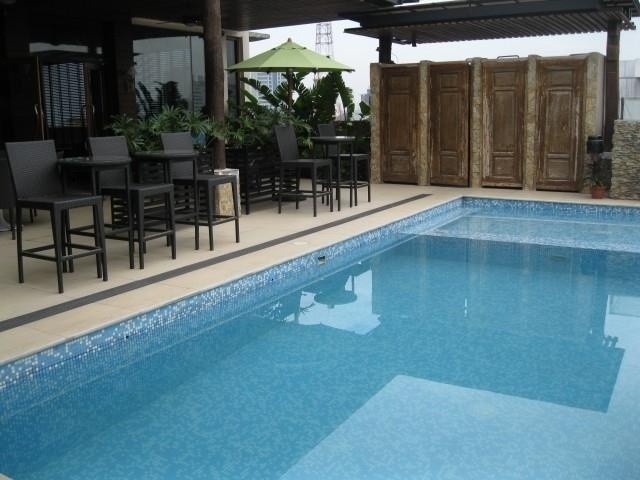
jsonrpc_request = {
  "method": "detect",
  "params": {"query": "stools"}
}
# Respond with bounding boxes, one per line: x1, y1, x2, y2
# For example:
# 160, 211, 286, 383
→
86, 137, 176, 271
6, 137, 105, 292
159, 132, 241, 252
195, 124, 370, 218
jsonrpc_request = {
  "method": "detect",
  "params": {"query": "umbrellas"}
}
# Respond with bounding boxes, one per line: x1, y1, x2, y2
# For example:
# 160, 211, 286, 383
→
223, 36, 356, 127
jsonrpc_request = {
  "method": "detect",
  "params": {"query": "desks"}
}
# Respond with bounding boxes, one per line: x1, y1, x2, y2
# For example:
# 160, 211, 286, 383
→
56, 155, 136, 276
134, 151, 197, 246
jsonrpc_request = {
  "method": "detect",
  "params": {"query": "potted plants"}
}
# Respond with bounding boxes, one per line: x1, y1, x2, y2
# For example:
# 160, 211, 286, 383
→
583, 156, 613, 199
